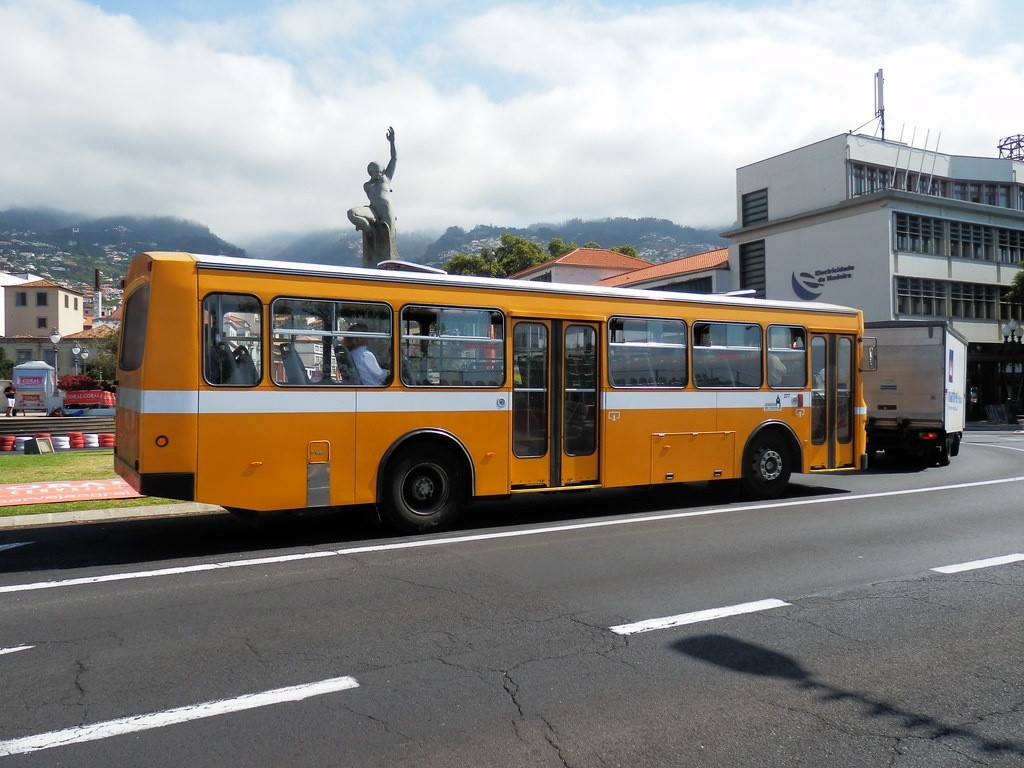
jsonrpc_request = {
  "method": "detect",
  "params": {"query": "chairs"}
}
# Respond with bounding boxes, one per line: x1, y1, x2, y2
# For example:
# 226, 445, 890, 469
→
389, 347, 417, 385
610, 353, 635, 386
218, 342, 244, 384
518, 355, 535, 398
629, 354, 656, 384
566, 354, 594, 402
232, 345, 259, 384
705, 358, 736, 386
280, 343, 310, 384
334, 346, 363, 386
534, 354, 545, 400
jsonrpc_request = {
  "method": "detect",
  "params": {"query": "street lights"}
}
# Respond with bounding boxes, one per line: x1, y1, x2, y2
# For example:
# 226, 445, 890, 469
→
71, 339, 82, 375
49, 327, 62, 396
80, 346, 90, 374
1002, 318, 1024, 424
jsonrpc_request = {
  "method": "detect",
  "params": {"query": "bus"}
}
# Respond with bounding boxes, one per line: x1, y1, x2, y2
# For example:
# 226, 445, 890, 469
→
113, 251, 879, 536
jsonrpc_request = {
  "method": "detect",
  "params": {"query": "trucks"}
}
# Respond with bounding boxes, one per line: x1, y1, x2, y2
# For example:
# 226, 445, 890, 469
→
863, 320, 969, 467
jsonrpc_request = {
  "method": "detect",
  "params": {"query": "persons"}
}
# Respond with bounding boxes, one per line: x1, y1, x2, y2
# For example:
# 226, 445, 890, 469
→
344, 324, 391, 385
347, 127, 398, 237
205, 324, 221, 385
816, 369, 825, 389
4, 382, 17, 417
70, 380, 116, 409
768, 344, 786, 386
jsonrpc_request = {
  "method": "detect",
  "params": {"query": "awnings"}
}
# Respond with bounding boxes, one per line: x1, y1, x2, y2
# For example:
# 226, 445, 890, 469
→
899, 319, 1004, 343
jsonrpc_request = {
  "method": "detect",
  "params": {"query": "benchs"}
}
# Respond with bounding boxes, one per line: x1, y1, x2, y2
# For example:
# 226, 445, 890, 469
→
12, 406, 52, 416
